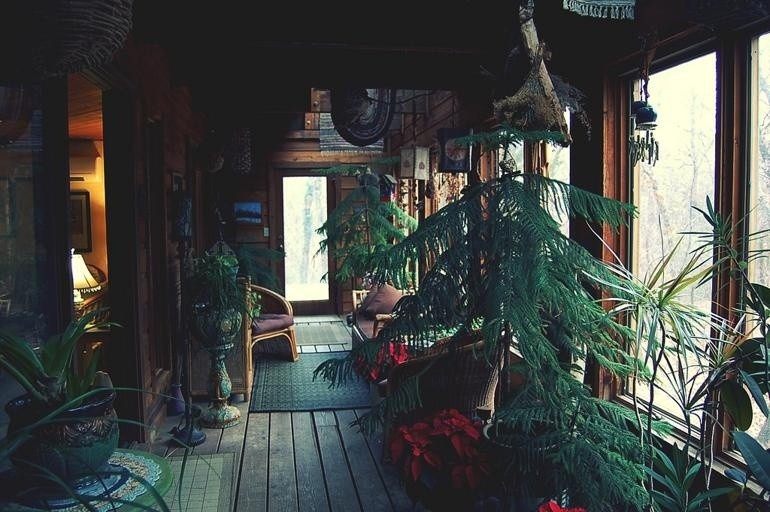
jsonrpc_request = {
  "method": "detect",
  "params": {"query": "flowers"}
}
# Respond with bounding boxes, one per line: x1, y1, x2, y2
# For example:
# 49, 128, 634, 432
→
390, 406, 494, 490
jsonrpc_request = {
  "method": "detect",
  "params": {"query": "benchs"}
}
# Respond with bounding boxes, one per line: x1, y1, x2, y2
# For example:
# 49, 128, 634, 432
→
351, 289, 433, 393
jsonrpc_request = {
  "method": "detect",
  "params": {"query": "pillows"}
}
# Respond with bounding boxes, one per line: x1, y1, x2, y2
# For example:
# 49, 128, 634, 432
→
358, 282, 404, 319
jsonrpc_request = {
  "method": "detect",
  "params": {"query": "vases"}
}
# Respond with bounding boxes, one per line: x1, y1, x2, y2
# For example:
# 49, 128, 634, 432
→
421, 458, 483, 512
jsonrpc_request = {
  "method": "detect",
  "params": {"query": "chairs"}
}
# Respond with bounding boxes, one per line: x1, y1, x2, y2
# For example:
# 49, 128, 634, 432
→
378, 323, 516, 465
248, 284, 299, 374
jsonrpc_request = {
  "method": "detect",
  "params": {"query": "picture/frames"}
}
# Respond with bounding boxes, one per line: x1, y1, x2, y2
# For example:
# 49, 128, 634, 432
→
231, 199, 264, 226
70, 192, 92, 254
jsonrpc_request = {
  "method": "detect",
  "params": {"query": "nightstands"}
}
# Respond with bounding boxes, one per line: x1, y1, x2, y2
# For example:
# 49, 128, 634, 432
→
0, 448, 173, 512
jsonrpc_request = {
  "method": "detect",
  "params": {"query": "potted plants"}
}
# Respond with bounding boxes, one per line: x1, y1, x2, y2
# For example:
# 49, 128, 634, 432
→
0, 305, 125, 490
169, 246, 259, 376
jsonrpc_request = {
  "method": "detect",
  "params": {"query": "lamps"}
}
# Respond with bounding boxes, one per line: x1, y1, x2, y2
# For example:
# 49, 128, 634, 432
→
169, 192, 206, 447
70, 254, 102, 303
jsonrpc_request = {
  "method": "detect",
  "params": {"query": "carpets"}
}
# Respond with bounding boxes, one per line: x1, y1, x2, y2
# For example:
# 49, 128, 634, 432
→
295, 324, 348, 346
141, 452, 236, 512
249, 350, 375, 413
